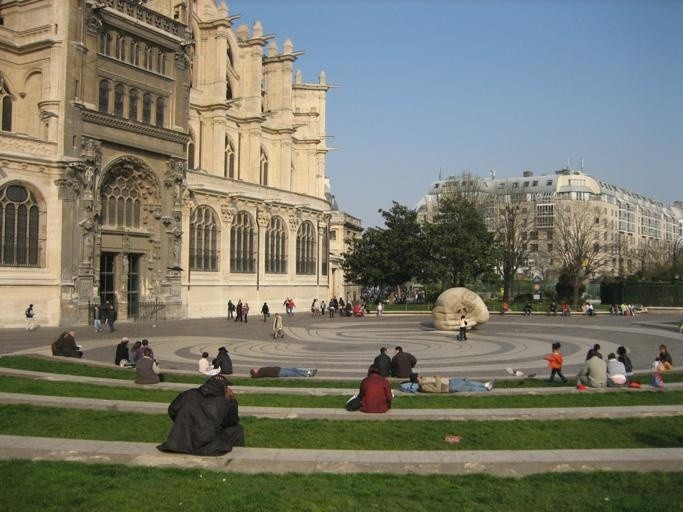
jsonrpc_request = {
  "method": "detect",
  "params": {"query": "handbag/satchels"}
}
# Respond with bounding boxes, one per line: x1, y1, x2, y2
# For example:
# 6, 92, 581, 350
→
345, 394, 361, 410
400, 381, 419, 393
608, 374, 626, 384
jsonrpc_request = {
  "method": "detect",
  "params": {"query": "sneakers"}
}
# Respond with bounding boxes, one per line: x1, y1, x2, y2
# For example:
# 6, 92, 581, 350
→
485, 379, 494, 391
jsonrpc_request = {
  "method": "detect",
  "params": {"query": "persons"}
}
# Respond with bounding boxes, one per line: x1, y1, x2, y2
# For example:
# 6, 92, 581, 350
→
410, 373, 497, 393
199, 352, 209, 374
544, 342, 673, 388
391, 346, 417, 378
375, 347, 392, 377
52, 301, 164, 385
228, 288, 425, 340
359, 365, 392, 413
250, 366, 317, 377
500, 301, 636, 318
459, 315, 467, 341
25, 304, 35, 332
156, 375, 244, 456
214, 347, 233, 374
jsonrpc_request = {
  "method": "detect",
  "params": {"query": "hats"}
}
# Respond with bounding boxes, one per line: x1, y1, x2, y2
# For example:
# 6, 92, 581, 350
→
212, 374, 232, 391
